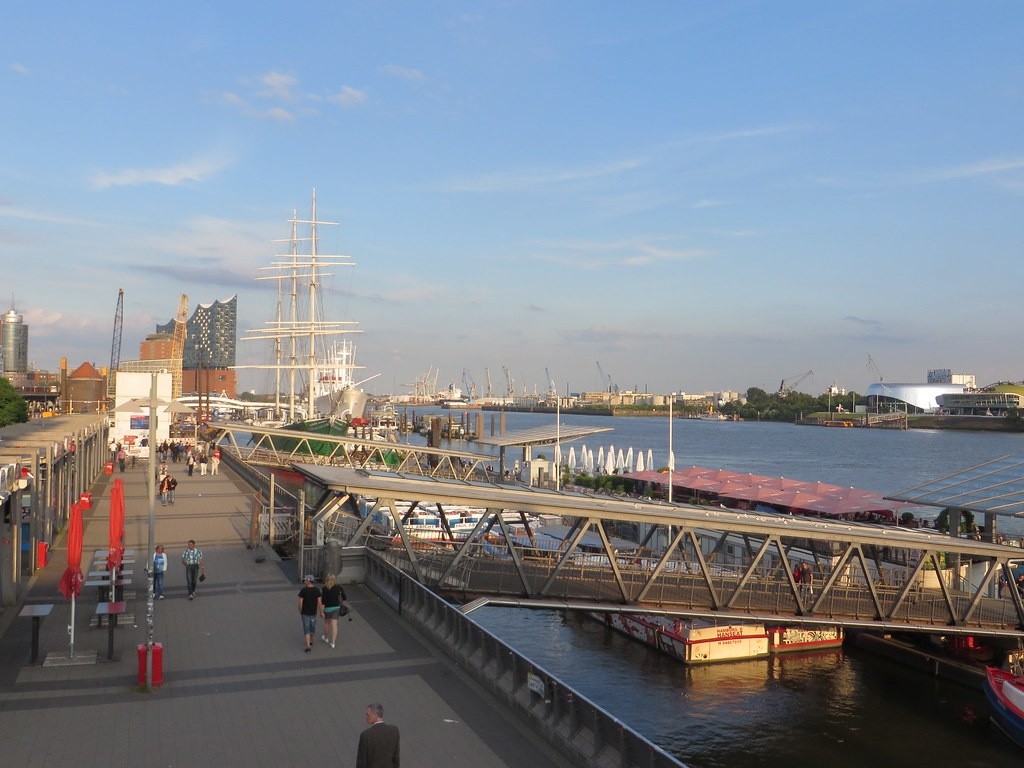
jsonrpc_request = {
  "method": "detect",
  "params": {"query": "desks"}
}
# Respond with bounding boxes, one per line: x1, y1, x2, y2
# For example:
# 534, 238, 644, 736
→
18, 545, 136, 662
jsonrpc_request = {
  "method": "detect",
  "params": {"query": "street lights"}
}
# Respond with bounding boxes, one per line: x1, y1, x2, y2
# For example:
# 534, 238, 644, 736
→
109, 371, 198, 692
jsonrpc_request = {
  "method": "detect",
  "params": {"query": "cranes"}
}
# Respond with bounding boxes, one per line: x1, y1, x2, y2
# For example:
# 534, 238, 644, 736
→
171, 293, 188, 397
596, 361, 614, 393
108, 287, 124, 387
778, 369, 814, 397
403, 364, 515, 404
545, 367, 555, 391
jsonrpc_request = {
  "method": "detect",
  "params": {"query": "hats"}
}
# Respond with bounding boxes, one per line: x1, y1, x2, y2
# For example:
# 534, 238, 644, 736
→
302, 574, 314, 582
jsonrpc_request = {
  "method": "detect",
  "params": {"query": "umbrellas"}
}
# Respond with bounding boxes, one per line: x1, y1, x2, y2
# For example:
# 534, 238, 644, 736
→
109, 478, 124, 604
60, 504, 83, 657
555, 444, 653, 477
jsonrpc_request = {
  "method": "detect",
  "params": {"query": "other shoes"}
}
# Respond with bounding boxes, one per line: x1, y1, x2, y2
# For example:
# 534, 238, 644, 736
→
159, 595, 164, 600
329, 643, 335, 648
310, 642, 312, 645
321, 635, 329, 643
188, 592, 196, 599
305, 648, 311, 653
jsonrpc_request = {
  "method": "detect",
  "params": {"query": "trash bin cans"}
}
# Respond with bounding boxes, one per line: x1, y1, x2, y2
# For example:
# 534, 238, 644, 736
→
136, 642, 164, 688
36, 541, 49, 568
79, 492, 92, 510
213, 449, 220, 461
104, 462, 114, 475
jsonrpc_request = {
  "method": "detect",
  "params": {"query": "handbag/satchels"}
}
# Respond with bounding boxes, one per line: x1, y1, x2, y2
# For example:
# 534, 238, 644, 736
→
339, 604, 353, 621
199, 574, 206, 582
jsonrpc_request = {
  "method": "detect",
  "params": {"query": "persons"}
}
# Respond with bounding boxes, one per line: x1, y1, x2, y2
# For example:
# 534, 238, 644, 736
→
793, 562, 813, 601
296, 574, 347, 654
182, 540, 203, 600
999, 575, 1024, 599
158, 439, 220, 476
146, 545, 168, 600
109, 442, 117, 460
116, 449, 126, 472
159, 475, 177, 506
356, 703, 400, 768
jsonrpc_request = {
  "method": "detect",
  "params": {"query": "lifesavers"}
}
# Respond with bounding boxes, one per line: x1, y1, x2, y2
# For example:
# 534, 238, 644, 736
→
672, 619, 682, 635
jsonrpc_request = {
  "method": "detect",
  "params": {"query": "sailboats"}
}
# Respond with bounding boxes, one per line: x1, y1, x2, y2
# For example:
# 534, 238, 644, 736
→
227, 185, 350, 457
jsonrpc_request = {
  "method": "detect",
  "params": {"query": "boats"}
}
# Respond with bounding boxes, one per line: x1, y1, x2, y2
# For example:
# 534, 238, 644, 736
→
302, 338, 369, 422
350, 491, 732, 572
978, 663, 1024, 749
